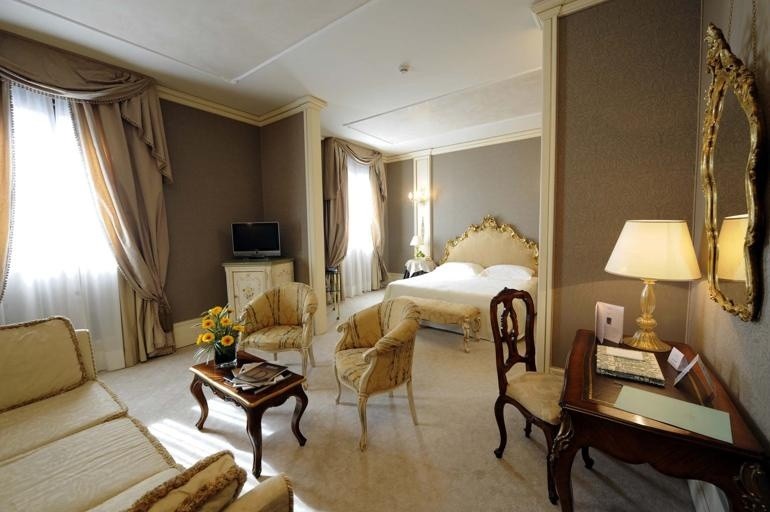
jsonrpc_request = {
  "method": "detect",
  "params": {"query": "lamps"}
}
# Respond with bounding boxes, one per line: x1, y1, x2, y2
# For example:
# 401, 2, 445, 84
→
604, 218, 702, 353
408, 187, 426, 205
409, 235, 425, 259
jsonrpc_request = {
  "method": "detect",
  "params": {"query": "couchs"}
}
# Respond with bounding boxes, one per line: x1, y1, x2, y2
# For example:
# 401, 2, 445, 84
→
237, 282, 320, 391
333, 297, 422, 452
0, 314, 295, 512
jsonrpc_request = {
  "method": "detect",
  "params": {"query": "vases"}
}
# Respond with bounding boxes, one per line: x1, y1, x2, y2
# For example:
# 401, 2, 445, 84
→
213, 343, 238, 369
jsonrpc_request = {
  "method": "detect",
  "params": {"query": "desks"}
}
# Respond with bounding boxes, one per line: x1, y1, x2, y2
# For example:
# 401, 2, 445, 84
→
548, 327, 769, 512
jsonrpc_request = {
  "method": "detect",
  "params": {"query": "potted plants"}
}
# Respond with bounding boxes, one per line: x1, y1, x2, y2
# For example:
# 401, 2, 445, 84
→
416, 251, 424, 260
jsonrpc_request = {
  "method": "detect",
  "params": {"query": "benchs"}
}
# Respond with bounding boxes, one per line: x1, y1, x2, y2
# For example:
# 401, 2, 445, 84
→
398, 295, 482, 353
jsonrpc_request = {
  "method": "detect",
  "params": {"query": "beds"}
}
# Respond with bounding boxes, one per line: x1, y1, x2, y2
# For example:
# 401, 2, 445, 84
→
382, 212, 539, 343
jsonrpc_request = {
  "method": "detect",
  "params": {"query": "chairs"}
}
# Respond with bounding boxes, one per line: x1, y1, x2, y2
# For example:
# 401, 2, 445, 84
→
489, 286, 596, 472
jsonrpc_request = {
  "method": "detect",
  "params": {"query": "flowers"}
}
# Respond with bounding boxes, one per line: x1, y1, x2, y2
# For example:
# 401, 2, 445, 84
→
190, 303, 245, 366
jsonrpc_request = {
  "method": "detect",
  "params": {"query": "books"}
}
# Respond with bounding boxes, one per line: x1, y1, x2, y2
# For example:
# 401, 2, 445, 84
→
223, 362, 293, 394
595, 301, 624, 344
596, 344, 666, 387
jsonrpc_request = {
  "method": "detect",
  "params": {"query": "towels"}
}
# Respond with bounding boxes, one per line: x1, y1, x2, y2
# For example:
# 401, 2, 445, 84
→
405, 258, 429, 278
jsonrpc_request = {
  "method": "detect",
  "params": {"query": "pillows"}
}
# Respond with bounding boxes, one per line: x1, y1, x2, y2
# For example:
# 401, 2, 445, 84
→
0, 317, 88, 413
123, 449, 248, 512
478, 264, 536, 281
435, 262, 485, 282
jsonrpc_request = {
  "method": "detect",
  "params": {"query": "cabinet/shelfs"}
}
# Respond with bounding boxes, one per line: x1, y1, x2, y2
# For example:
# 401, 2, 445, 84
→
220, 257, 297, 322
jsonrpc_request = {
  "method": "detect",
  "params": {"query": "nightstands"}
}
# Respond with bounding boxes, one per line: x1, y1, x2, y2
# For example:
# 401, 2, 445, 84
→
403, 259, 434, 279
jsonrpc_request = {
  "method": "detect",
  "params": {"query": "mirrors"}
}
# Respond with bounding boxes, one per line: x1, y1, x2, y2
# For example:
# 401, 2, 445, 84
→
701, 21, 764, 326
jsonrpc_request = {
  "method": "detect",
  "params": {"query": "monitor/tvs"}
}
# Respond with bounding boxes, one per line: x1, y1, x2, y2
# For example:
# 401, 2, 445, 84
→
231, 221, 281, 261
